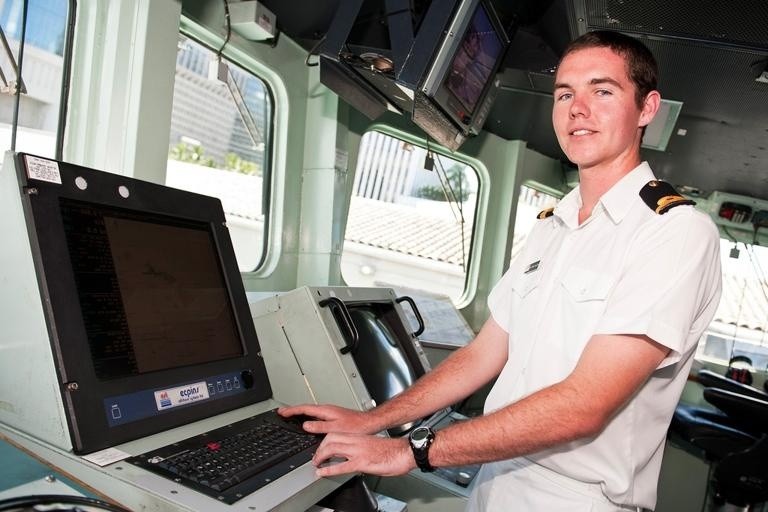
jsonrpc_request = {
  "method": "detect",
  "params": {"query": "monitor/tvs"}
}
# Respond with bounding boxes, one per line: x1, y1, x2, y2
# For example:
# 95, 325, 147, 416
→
328, 299, 444, 438
19, 152, 276, 456
336, 0, 512, 137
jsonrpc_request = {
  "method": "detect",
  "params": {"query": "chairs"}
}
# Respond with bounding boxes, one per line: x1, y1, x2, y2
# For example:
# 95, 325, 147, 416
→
667, 370, 767, 510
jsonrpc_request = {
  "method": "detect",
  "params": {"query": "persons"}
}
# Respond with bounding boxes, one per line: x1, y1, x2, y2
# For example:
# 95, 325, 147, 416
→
274, 29, 726, 512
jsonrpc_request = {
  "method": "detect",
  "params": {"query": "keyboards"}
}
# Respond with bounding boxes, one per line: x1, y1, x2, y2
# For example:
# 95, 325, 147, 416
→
126, 406, 326, 505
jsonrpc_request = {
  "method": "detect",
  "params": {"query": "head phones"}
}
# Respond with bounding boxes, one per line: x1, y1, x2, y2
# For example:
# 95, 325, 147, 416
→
725, 355, 753, 386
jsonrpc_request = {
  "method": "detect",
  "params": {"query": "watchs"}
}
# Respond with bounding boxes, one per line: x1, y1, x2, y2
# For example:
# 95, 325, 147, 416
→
408, 423, 439, 472
